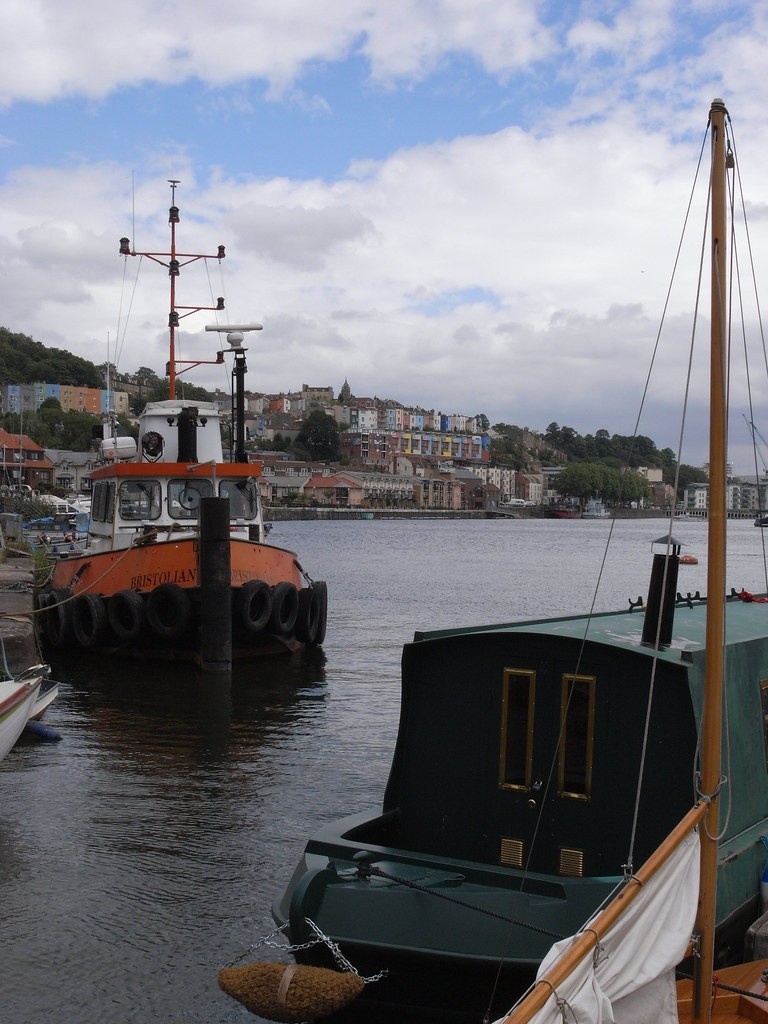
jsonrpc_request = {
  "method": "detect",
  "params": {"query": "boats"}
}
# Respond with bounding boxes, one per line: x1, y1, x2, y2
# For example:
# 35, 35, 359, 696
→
271, 591, 766, 1023
543, 502, 579, 519
581, 489, 611, 519
1, 178, 328, 765
673, 512, 689, 520
754, 513, 768, 527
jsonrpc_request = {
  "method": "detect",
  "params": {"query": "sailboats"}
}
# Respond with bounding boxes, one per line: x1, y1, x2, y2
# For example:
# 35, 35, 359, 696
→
481, 92, 766, 1024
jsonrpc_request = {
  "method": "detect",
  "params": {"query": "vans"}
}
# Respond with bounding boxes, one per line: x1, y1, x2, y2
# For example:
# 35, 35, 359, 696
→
510, 498, 526, 507
